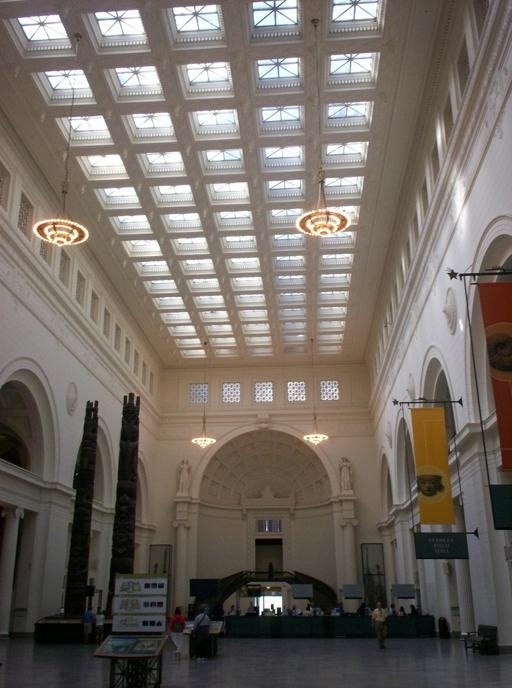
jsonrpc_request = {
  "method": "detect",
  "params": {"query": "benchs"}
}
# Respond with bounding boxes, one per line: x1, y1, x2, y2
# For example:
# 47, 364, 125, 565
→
460, 624, 500, 655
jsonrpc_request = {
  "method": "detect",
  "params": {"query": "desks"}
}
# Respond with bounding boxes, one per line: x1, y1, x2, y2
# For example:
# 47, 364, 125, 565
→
224, 615, 437, 639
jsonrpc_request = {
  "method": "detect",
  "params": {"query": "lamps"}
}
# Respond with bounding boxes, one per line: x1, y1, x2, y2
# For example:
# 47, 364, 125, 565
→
302, 338, 328, 446
32, 33, 89, 248
294, 18, 352, 239
191, 342, 217, 450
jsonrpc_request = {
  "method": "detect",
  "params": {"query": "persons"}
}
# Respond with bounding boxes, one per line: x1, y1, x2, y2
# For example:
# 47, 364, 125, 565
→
371, 600, 388, 650
227, 599, 423, 616
95, 607, 105, 647
82, 607, 94, 646
168, 607, 186, 657
416, 474, 446, 498
192, 603, 211, 662
